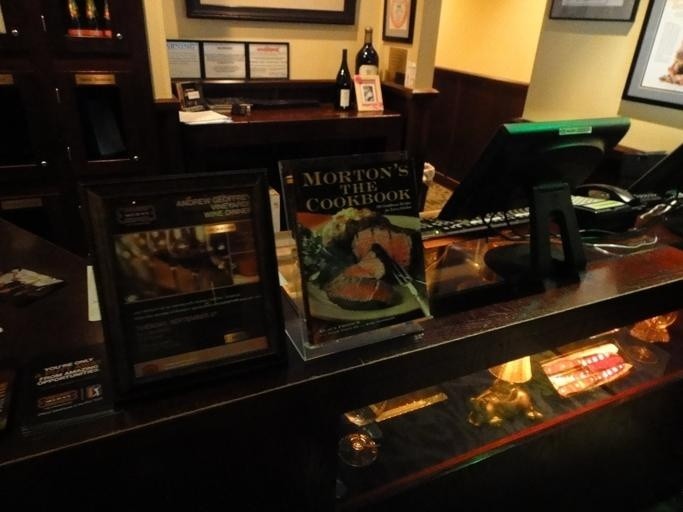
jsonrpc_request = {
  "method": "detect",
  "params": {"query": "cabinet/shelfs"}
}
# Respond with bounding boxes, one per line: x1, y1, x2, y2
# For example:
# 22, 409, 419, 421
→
0, 0, 163, 256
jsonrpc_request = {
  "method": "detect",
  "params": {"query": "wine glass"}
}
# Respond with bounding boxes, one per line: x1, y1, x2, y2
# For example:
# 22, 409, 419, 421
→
133, 222, 237, 294
628, 312, 677, 363
336, 401, 386, 468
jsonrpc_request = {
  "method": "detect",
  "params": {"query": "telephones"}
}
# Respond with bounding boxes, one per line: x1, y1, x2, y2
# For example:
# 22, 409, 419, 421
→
570, 183, 640, 233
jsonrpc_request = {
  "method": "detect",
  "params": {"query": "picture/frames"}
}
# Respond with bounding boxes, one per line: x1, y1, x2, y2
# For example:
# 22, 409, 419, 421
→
185, 0, 357, 26
381, 1, 417, 44
548, 1, 640, 23
617, 2, 683, 112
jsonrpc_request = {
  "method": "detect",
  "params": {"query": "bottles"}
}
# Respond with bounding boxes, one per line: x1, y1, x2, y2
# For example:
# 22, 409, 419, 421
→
355, 28, 379, 76
335, 48, 353, 112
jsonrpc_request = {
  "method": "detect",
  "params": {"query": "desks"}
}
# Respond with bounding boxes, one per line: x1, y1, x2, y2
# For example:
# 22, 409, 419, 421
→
179, 96, 405, 173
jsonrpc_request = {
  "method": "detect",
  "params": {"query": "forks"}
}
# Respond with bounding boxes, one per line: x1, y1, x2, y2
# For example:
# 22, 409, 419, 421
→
394, 266, 434, 322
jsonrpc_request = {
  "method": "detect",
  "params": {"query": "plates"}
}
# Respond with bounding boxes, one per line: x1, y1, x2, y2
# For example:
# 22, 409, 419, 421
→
308, 210, 427, 322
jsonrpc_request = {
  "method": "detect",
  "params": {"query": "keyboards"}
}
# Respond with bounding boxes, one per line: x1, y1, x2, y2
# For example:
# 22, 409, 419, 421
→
632, 192, 662, 204
422, 207, 530, 236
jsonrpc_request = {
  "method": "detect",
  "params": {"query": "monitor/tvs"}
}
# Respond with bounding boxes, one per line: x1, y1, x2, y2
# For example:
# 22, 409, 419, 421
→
435, 115, 631, 279
629, 143, 683, 197
